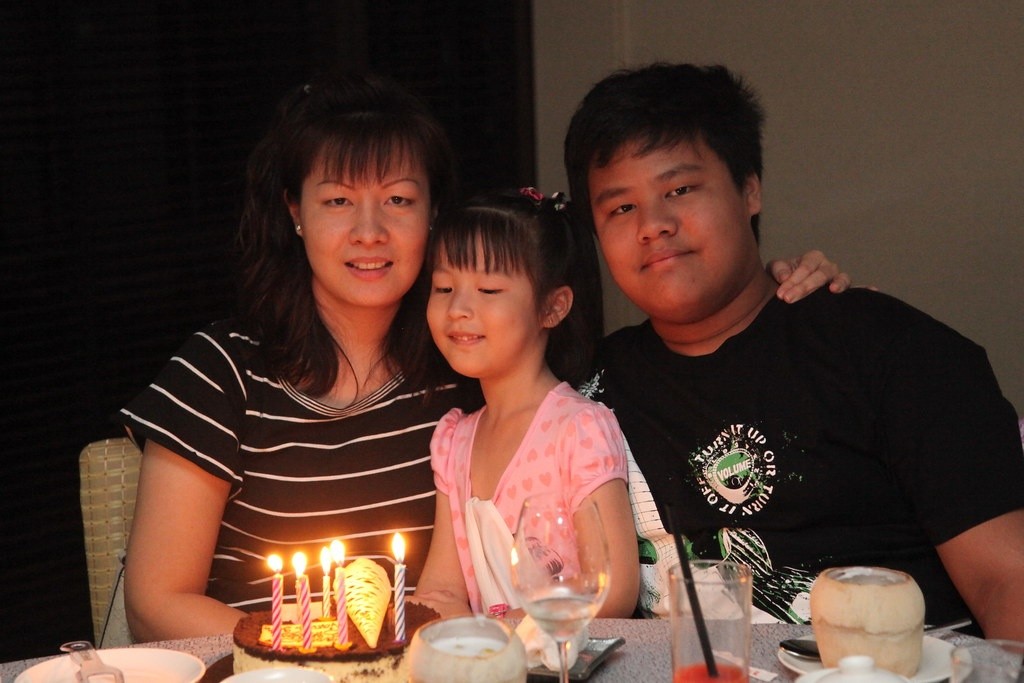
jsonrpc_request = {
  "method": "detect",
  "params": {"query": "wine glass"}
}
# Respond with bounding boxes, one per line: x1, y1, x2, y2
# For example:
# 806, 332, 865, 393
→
510, 490, 613, 683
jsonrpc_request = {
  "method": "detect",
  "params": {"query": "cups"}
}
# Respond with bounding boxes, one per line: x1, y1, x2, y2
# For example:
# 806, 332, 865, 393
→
951, 639, 1024, 683
794, 655, 912, 683
667, 560, 753, 682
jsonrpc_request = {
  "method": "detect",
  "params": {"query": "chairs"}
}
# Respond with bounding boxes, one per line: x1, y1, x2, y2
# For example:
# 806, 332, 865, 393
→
79, 437, 144, 649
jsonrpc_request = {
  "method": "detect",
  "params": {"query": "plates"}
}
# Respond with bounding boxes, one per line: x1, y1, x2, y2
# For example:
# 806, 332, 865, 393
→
219, 668, 335, 683
13, 648, 206, 683
778, 635, 975, 683
526, 636, 626, 682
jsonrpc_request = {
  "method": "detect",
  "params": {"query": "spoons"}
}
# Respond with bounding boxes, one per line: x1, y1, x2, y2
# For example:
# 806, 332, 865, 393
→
779, 616, 973, 660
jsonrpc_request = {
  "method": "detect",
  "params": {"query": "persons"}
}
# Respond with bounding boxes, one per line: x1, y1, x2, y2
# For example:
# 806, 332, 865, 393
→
555, 60, 1024, 677
117, 60, 881, 651
392, 181, 641, 628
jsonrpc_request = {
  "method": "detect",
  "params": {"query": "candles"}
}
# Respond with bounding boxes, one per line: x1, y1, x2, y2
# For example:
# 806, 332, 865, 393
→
267, 554, 284, 650
294, 552, 313, 650
331, 540, 348, 645
392, 533, 406, 642
321, 548, 332, 618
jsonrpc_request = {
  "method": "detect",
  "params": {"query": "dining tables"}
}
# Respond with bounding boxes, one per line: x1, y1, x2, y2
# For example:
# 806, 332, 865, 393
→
1, 619, 1024, 682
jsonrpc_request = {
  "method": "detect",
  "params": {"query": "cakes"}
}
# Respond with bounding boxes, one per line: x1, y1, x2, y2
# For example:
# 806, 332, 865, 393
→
233, 602, 441, 683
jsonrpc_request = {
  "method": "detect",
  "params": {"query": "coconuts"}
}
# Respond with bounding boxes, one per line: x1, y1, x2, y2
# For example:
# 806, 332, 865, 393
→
810, 567, 926, 679
